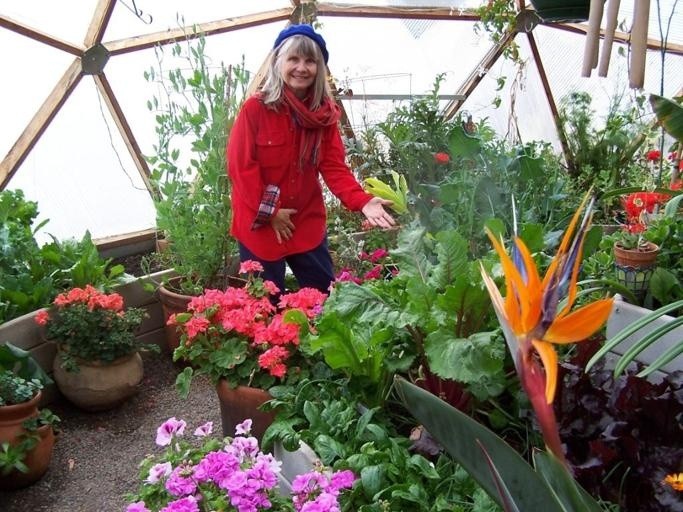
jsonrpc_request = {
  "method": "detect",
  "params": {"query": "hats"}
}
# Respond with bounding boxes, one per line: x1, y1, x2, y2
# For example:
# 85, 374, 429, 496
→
274, 24, 328, 66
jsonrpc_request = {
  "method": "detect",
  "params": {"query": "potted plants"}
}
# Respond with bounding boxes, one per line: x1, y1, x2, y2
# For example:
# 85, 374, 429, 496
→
0, 370, 61, 491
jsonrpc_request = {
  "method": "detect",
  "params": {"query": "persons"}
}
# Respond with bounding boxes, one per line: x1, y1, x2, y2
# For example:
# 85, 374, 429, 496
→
223, 25, 394, 319
